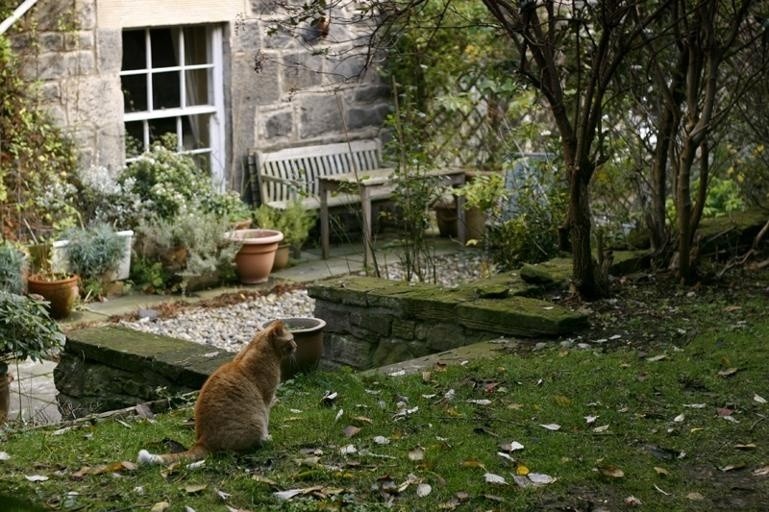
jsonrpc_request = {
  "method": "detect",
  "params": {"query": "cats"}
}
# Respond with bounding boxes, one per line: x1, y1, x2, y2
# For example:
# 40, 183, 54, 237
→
137, 317, 298, 468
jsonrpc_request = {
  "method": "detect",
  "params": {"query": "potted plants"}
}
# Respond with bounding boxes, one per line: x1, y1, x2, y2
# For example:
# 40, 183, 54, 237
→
0, 289, 64, 424
0, 144, 316, 318
455, 173, 505, 241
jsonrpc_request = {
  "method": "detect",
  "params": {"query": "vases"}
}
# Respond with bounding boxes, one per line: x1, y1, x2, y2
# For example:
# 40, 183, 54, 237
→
259, 316, 326, 381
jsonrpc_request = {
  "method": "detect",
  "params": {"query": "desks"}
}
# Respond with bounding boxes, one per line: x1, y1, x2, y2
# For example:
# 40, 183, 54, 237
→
318, 162, 469, 267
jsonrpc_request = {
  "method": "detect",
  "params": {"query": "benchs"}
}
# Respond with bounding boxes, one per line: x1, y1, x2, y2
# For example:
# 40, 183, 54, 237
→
254, 137, 424, 257
